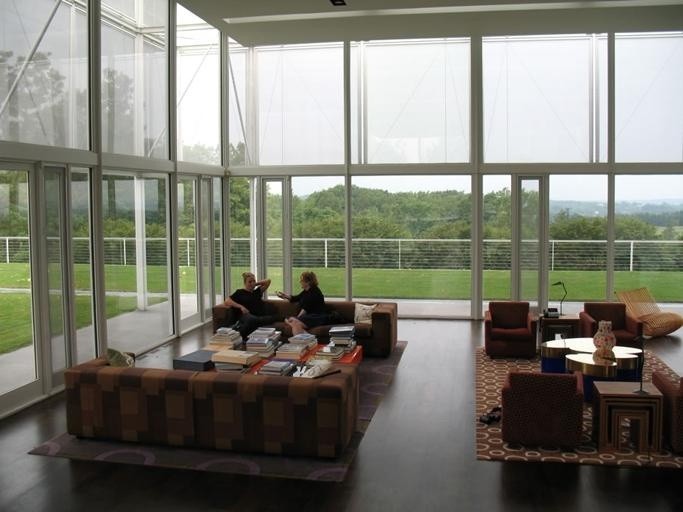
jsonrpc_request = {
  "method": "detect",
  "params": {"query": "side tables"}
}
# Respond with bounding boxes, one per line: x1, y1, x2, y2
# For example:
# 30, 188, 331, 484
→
540, 313, 663, 454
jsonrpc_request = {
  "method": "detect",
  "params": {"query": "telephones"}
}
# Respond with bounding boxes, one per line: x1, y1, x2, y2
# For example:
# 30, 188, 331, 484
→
543, 308, 559, 317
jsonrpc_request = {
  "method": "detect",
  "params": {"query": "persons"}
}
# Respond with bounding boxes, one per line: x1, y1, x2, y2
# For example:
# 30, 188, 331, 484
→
275, 272, 326, 343
224, 271, 279, 323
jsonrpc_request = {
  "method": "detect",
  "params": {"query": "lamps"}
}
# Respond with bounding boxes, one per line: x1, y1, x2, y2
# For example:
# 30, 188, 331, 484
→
551, 280, 567, 316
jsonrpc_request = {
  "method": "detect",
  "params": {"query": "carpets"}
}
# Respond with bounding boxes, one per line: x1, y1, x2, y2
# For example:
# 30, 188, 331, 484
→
473, 345, 683, 467
25, 339, 407, 482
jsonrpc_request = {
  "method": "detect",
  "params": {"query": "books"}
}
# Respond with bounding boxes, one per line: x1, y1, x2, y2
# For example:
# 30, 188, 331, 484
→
206, 326, 360, 380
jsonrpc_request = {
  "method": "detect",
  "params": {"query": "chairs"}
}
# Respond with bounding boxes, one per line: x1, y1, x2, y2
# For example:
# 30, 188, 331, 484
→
652, 371, 683, 456
482, 301, 537, 360
579, 286, 683, 348
500, 366, 584, 450
